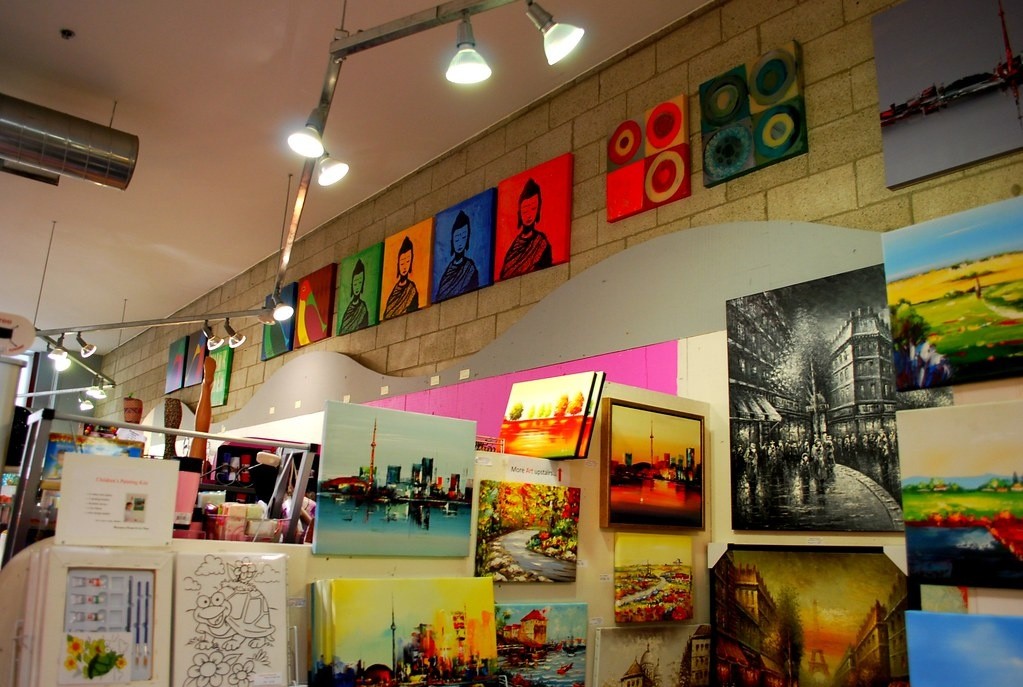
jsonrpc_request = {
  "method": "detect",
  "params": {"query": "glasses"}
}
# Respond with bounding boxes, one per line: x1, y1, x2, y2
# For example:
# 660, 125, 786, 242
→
365, 679, 391, 687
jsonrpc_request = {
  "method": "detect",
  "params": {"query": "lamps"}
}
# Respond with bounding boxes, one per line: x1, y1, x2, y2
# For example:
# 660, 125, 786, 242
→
524, 3, 584, 66
256, 314, 276, 325
317, 147, 348, 186
76, 337, 97, 359
223, 323, 246, 349
272, 296, 294, 321
288, 107, 327, 158
201, 325, 224, 351
92, 376, 107, 400
86, 375, 101, 396
78, 394, 94, 411
47, 338, 68, 360
445, 22, 491, 84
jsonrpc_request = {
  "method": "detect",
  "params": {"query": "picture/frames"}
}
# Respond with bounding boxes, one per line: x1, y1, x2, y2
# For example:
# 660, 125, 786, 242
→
37, 547, 173, 687
706, 541, 921, 687
598, 397, 706, 533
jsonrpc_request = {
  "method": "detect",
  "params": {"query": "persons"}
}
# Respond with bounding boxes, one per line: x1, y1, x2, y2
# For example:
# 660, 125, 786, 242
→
124, 502, 137, 522
188, 356, 217, 461
